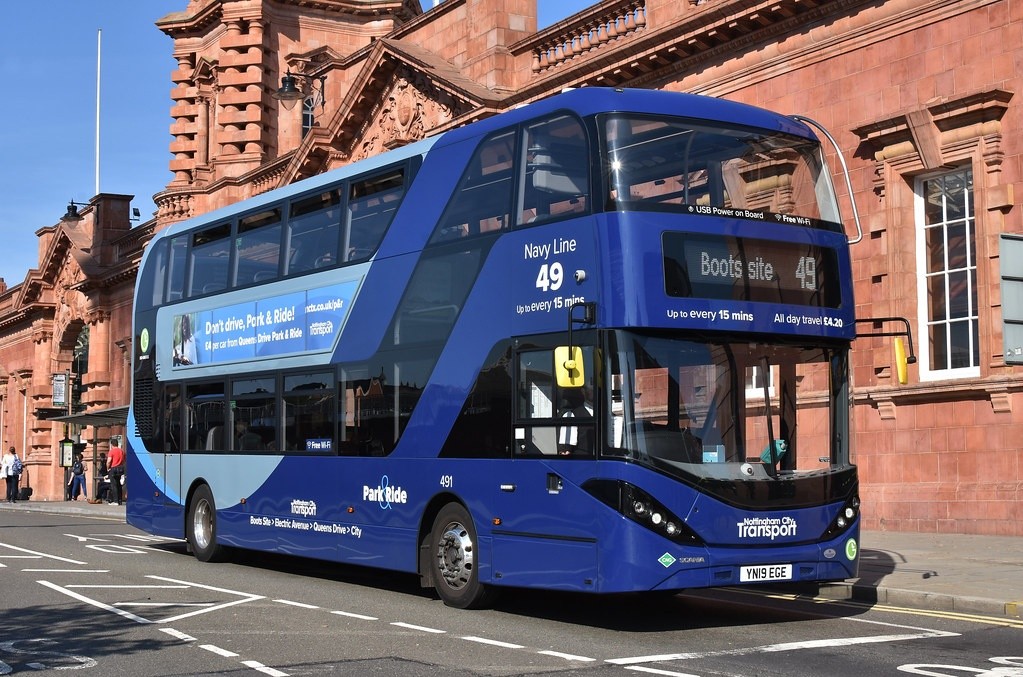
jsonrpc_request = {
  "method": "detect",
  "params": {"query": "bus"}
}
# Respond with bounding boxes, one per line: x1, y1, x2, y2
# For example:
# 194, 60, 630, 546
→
123, 86, 917, 610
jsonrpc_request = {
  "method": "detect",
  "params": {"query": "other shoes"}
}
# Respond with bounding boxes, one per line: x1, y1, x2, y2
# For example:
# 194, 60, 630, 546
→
6, 499, 16, 503
84, 498, 88, 502
71, 498, 75, 501
108, 502, 122, 505
89, 498, 102, 504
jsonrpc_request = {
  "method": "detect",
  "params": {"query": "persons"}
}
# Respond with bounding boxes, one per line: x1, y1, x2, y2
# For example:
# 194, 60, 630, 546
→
558, 372, 606, 458
106, 439, 126, 505
68, 454, 89, 501
88, 466, 125, 504
97, 453, 107, 487
1, 446, 23, 504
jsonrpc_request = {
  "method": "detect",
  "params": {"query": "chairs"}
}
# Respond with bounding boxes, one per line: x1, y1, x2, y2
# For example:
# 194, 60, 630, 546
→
164, 205, 564, 300
107, 478, 126, 503
206, 425, 264, 452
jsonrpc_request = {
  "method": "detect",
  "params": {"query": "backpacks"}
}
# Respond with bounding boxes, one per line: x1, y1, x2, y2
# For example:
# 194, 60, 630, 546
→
12, 454, 22, 476
98, 459, 108, 476
74, 460, 83, 475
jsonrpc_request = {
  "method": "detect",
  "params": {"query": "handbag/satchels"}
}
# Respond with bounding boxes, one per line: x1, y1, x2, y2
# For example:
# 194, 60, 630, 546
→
0, 464, 8, 479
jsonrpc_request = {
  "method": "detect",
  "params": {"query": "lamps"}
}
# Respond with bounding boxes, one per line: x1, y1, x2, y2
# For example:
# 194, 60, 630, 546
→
273, 68, 326, 111
